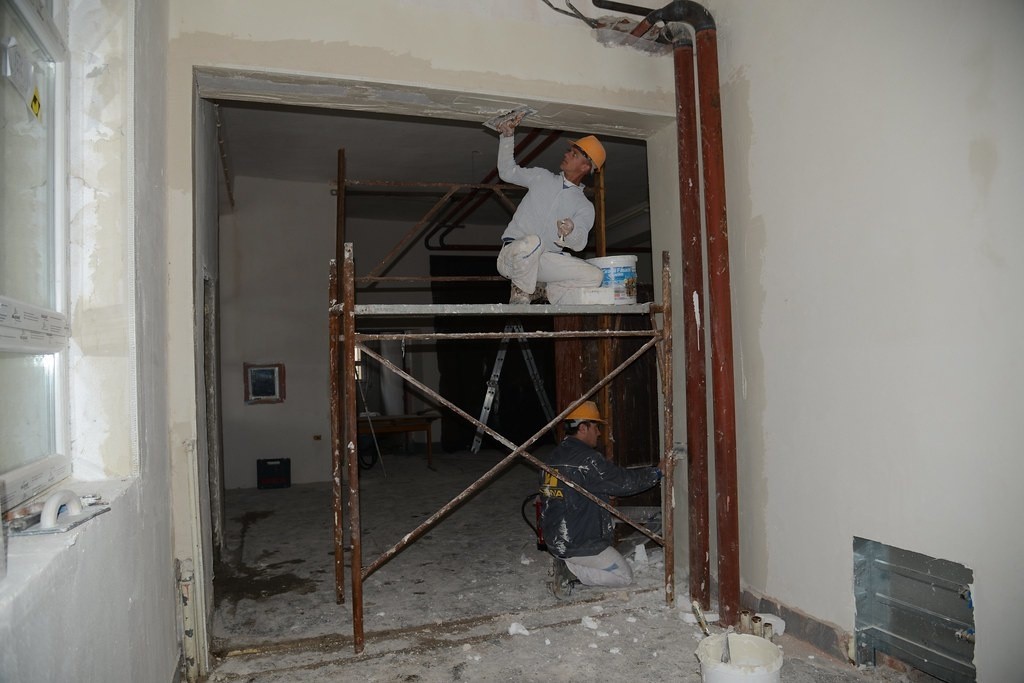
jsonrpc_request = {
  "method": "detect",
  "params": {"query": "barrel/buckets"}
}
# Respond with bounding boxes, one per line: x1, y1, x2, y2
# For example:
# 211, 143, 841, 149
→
700, 634, 781, 683
585, 256, 638, 304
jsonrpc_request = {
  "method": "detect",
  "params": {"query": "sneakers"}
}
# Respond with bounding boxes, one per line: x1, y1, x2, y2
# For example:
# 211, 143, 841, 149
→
529, 281, 548, 303
544, 556, 576, 601
509, 281, 531, 305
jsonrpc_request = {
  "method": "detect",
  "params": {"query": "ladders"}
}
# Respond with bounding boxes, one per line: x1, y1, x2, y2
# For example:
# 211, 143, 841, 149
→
471, 320, 561, 456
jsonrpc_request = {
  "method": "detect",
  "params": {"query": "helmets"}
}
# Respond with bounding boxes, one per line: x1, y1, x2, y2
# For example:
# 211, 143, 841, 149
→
568, 135, 606, 173
563, 401, 608, 425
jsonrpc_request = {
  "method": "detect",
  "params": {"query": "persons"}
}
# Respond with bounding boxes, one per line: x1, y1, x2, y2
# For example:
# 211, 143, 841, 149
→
496, 118, 607, 304
538, 401, 667, 601
